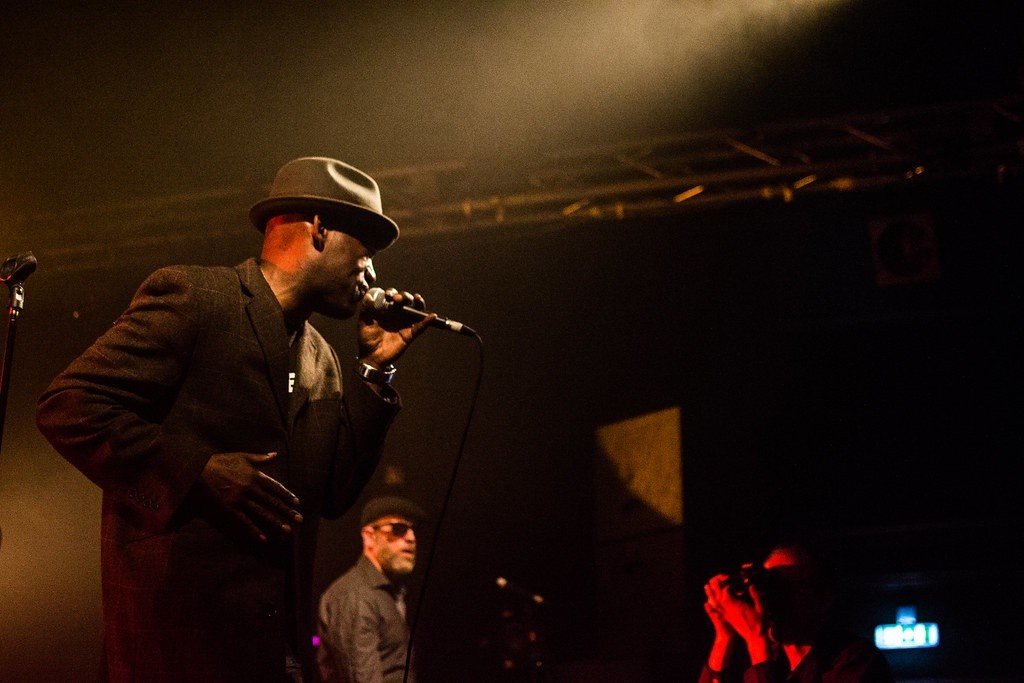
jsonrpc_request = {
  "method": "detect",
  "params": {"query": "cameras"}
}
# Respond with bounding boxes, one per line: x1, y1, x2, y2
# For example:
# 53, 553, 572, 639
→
723, 565, 783, 602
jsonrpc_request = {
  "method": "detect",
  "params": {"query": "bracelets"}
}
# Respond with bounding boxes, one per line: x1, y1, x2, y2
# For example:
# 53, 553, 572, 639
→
352, 356, 397, 386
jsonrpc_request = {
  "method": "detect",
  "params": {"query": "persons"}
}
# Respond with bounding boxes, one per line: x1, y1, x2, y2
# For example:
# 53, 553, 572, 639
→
690, 538, 894, 683
313, 493, 424, 683
26, 152, 444, 683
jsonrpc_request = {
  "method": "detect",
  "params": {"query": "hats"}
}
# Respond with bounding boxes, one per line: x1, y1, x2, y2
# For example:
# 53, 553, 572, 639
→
361, 495, 428, 529
247, 156, 400, 253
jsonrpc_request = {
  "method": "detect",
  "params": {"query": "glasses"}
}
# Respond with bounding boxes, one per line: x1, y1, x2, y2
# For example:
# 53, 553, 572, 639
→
372, 521, 420, 538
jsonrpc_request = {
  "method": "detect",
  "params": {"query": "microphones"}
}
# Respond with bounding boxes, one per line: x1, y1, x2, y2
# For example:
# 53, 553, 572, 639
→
363, 288, 478, 338
